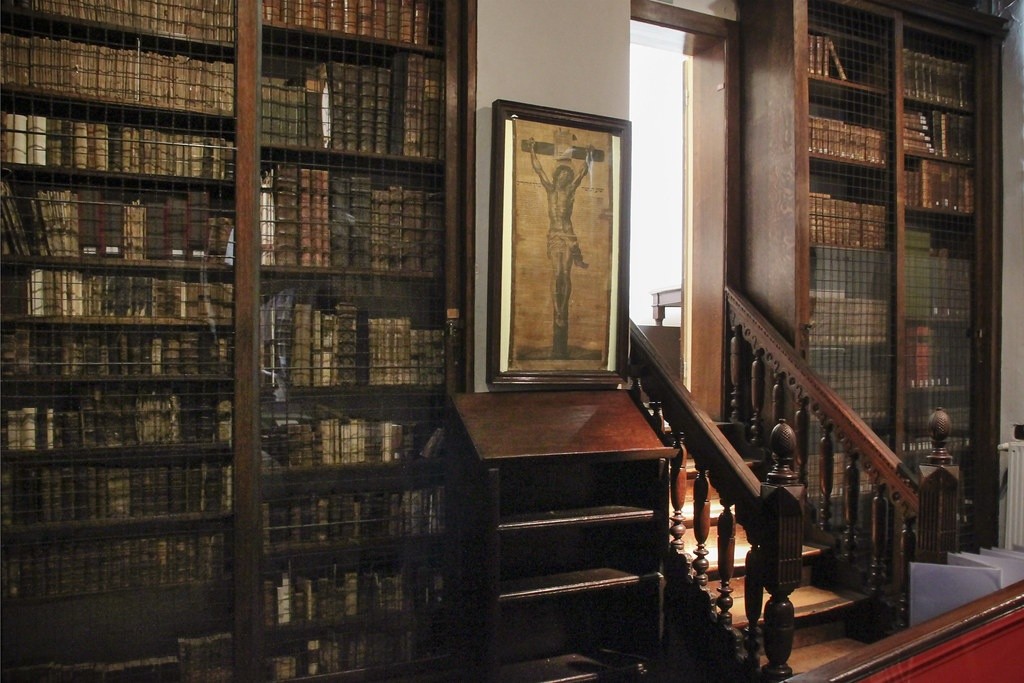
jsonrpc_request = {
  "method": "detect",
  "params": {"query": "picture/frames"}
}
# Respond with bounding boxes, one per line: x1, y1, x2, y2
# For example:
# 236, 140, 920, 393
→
484, 98, 631, 393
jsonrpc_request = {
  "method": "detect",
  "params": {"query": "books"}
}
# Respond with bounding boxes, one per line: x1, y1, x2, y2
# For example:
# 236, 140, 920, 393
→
1, 0, 446, 683
805, 34, 974, 499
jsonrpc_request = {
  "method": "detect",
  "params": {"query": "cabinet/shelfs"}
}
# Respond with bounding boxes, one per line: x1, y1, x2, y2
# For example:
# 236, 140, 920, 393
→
445, 389, 682, 683
0, 0, 476, 683
739, 0, 1010, 561
